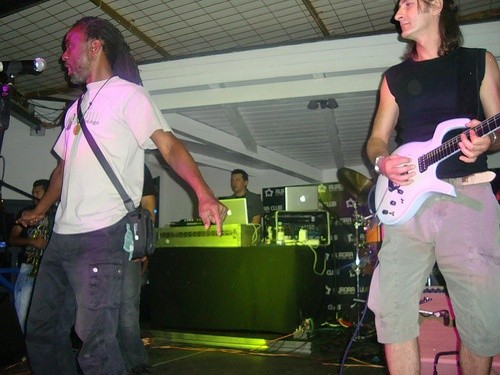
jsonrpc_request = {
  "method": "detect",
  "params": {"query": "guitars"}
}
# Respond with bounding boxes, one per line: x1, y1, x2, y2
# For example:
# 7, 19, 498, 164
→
374, 113, 500, 226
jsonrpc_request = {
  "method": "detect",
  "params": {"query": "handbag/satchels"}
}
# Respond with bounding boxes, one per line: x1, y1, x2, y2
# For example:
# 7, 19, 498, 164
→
126, 208, 155, 259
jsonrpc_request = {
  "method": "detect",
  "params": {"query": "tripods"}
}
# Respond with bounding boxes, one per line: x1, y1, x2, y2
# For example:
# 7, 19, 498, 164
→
329, 199, 376, 341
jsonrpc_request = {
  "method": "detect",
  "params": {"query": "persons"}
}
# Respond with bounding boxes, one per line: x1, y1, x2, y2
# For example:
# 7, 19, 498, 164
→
4, 179, 58, 371
121, 163, 154, 375
366, 0, 500, 375
15, 17, 228, 375
230, 169, 264, 224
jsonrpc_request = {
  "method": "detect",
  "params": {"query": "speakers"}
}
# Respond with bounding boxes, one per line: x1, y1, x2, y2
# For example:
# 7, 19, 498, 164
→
417, 286, 500, 365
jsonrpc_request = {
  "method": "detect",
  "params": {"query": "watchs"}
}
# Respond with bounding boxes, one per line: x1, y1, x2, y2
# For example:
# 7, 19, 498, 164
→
374, 156, 383, 172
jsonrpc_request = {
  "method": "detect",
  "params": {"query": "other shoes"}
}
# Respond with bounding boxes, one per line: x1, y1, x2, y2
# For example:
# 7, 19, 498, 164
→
132, 365, 152, 375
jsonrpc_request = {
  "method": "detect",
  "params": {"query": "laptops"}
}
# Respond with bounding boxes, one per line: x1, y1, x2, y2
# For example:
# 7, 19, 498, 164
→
285, 186, 320, 211
216, 198, 260, 226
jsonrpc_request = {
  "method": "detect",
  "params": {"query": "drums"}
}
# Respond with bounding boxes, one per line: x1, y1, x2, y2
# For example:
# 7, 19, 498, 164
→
366, 223, 381, 248
417, 286, 500, 374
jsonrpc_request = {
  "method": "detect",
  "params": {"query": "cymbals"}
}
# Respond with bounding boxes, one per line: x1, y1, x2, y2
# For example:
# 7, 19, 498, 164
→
337, 167, 375, 210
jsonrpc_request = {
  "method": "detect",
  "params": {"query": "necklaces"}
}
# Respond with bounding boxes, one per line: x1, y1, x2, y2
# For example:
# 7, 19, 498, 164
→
72, 76, 112, 135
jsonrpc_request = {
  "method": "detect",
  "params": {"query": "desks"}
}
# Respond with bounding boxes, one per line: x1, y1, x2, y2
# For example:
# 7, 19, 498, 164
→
140, 244, 327, 334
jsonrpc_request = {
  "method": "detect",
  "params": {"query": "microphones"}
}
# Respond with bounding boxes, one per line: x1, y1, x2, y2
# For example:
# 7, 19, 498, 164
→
0, 57, 47, 72
441, 309, 450, 326
